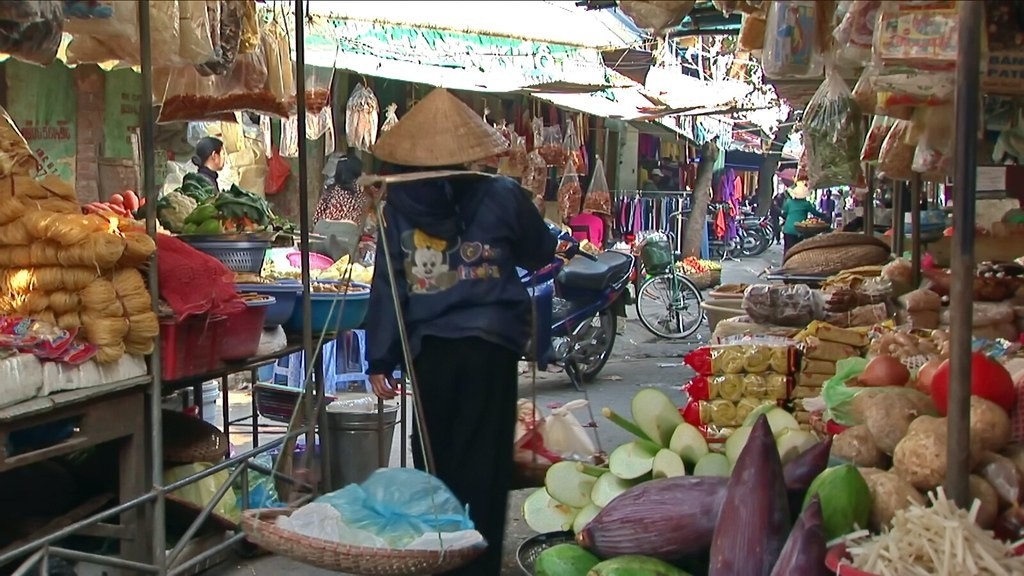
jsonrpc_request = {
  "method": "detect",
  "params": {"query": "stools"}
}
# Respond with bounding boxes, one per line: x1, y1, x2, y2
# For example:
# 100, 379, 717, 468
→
270, 328, 401, 396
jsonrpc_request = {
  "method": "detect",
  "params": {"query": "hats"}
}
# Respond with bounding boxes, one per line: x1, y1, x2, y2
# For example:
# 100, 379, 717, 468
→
793, 185, 809, 199
372, 88, 513, 167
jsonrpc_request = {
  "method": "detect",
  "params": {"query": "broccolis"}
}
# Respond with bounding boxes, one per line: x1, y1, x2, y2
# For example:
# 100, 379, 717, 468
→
154, 190, 199, 232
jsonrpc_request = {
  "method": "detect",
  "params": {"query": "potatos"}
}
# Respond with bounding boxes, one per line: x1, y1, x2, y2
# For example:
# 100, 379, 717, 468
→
826, 387, 1024, 542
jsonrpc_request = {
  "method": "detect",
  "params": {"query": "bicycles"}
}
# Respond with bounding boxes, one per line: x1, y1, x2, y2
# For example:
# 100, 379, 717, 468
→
631, 208, 707, 339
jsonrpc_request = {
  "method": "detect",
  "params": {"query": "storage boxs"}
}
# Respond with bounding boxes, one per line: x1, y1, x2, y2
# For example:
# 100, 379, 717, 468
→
158, 315, 230, 381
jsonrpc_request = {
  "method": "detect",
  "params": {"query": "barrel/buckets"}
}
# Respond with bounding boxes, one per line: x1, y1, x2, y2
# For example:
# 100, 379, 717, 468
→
324, 404, 399, 492
157, 376, 219, 430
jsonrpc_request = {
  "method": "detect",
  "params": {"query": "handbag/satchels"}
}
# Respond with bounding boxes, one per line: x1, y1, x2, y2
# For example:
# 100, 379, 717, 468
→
263, 143, 291, 194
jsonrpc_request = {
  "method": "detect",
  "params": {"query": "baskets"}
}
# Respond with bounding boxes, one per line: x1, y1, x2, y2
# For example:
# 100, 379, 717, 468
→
183, 241, 272, 274
511, 453, 610, 491
241, 506, 486, 576
927, 268, 1024, 302
781, 232, 891, 284
641, 242, 672, 276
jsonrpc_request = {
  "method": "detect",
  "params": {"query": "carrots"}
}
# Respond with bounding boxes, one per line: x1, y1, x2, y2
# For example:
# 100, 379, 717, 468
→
82, 190, 146, 221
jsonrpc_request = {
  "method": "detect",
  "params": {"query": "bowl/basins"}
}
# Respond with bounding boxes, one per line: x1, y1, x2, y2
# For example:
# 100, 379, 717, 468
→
250, 381, 337, 427
212, 276, 371, 360
709, 290, 745, 299
286, 251, 334, 270
700, 298, 747, 331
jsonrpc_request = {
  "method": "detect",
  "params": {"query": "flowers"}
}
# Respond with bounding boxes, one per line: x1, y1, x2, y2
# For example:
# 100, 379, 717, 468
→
667, 255, 722, 276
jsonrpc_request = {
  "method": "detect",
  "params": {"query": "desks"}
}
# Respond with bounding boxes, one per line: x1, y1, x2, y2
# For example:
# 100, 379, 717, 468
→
0, 373, 153, 576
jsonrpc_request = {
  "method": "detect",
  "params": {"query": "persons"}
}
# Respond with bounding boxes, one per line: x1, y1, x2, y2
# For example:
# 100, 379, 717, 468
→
365, 90, 559, 576
192, 136, 225, 193
311, 155, 366, 230
748, 184, 835, 256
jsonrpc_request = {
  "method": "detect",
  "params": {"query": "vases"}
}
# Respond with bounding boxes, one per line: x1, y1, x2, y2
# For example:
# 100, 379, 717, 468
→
710, 269, 721, 288
679, 271, 710, 291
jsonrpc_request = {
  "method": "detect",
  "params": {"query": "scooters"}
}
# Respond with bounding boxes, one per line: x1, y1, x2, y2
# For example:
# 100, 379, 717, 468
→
704, 202, 776, 264
515, 217, 646, 384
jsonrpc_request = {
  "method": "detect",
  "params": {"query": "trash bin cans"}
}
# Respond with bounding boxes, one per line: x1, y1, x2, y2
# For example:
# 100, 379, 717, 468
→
324, 403, 398, 494
